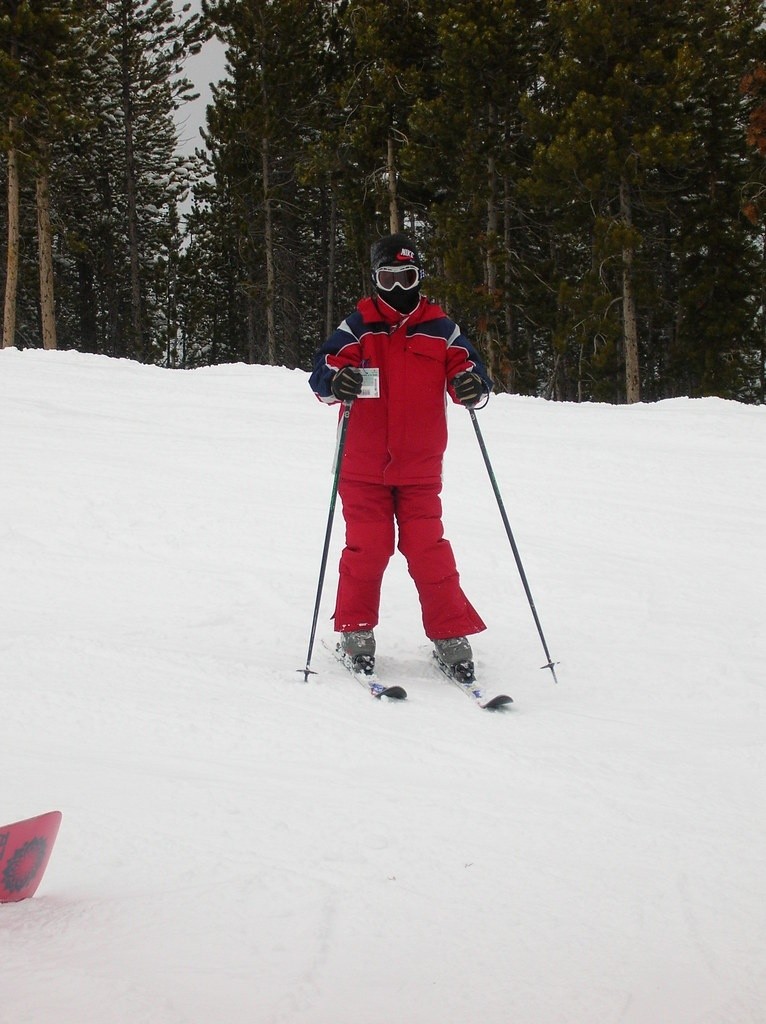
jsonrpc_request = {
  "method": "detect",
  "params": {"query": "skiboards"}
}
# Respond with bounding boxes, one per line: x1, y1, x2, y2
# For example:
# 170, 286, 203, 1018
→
318, 634, 515, 710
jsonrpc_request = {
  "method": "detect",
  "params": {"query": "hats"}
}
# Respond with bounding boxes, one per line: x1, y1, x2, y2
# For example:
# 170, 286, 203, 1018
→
370, 233, 421, 270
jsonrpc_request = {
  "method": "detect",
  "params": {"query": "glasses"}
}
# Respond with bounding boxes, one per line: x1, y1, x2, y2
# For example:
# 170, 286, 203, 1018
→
370, 264, 425, 292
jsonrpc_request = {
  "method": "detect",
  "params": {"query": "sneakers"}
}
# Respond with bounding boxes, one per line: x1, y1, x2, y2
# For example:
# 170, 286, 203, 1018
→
433, 635, 478, 686
336, 628, 376, 675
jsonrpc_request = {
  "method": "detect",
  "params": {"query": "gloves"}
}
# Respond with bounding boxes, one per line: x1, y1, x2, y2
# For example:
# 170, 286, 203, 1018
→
449, 371, 482, 403
329, 364, 363, 400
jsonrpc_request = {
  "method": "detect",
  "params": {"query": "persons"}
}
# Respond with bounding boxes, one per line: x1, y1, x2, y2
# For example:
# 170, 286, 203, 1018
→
309, 233, 494, 667
